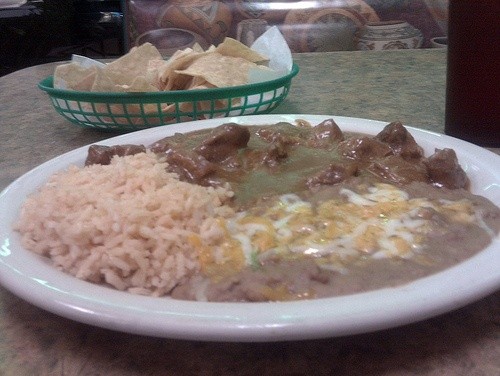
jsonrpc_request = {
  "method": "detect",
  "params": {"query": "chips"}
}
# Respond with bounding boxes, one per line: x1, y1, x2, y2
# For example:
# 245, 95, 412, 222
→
52, 36, 272, 130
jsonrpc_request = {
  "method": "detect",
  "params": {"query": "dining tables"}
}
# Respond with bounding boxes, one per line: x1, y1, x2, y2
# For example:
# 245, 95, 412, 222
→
0, 45, 500, 375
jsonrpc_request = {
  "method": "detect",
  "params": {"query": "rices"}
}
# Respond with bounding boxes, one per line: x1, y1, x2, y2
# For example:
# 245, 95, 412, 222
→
14, 150, 234, 298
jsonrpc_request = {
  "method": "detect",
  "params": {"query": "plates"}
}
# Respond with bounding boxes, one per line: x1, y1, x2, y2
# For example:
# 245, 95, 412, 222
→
0, 114, 500, 344
